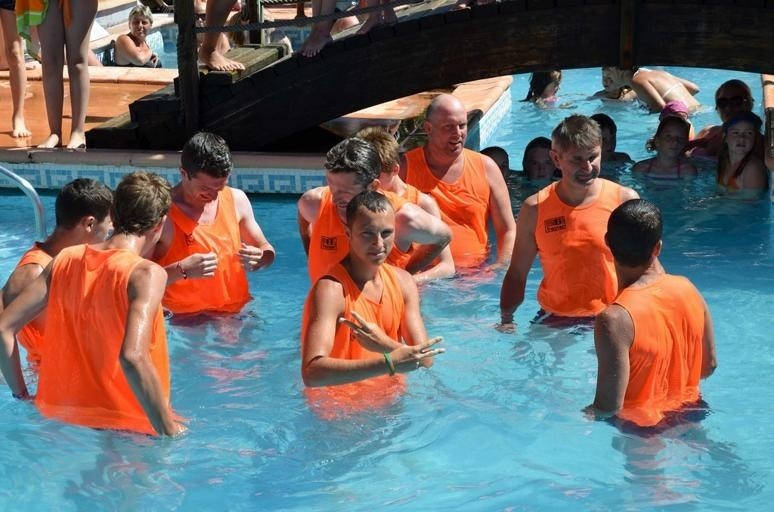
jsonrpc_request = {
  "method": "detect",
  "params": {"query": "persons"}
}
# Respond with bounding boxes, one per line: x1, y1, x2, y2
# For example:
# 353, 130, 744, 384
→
397, 93, 517, 276
580, 199, 719, 443
498, 113, 665, 339
142, 131, 276, 329
299, 191, 446, 424
0, 170, 190, 447
0, 0, 472, 155
0, 176, 116, 383
478, 66, 774, 192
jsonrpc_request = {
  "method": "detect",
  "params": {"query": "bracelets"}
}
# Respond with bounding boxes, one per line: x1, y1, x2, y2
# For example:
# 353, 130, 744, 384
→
176, 259, 187, 279
384, 353, 399, 379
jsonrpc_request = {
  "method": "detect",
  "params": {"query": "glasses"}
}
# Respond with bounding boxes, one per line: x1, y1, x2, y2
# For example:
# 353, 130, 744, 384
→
193, 13, 206, 21
717, 95, 748, 109
